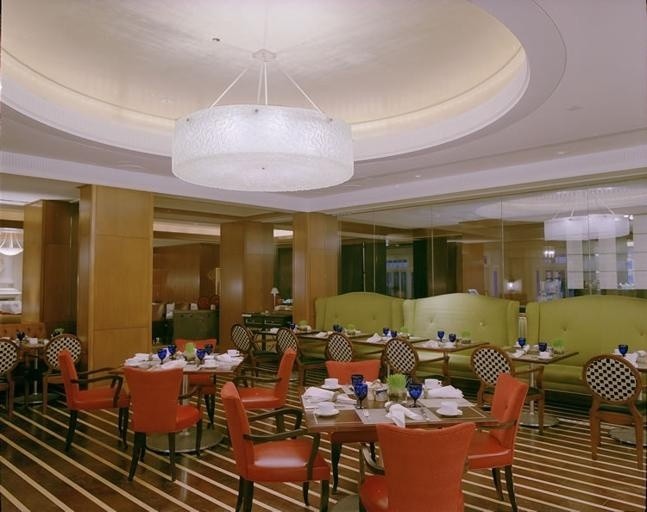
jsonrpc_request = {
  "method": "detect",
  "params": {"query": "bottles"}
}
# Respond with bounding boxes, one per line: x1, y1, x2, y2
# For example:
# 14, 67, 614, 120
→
365, 382, 388, 402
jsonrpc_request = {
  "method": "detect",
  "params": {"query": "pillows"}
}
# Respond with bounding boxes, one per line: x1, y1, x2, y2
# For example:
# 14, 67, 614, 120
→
175, 302, 190, 310
152, 302, 163, 320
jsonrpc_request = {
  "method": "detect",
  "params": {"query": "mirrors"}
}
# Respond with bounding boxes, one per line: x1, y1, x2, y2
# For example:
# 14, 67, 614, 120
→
338, 178, 647, 306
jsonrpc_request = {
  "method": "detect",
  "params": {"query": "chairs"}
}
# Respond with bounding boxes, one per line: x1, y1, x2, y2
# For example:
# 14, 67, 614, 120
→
470, 344, 546, 434
583, 354, 646, 470
125, 338, 229, 480
230, 324, 330, 389
1, 336, 20, 419
39, 335, 130, 453
446, 374, 528, 512
358, 421, 476, 512
219, 348, 342, 511
322, 332, 420, 388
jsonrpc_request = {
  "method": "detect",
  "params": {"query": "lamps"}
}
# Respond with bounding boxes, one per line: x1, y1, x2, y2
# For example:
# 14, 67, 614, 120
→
270, 288, 279, 310
172, 49, 355, 193
0, 233, 24, 256
544, 190, 630, 242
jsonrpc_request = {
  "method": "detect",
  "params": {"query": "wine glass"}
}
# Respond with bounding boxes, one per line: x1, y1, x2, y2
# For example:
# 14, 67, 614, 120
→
407, 383, 423, 408
618, 343, 628, 359
16, 331, 61, 345
351, 374, 367, 410
438, 331, 458, 344
383, 327, 408, 341
154, 343, 216, 366
332, 323, 355, 335
517, 337, 547, 354
283, 320, 297, 332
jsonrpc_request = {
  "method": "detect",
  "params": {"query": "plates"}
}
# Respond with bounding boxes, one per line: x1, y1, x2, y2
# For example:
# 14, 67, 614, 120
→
423, 384, 444, 391
436, 408, 463, 417
313, 410, 339, 416
319, 385, 342, 390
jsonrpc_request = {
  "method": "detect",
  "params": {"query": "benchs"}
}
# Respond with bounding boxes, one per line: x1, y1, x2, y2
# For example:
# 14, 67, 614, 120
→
516, 295, 647, 403
301, 293, 404, 362
402, 292, 520, 382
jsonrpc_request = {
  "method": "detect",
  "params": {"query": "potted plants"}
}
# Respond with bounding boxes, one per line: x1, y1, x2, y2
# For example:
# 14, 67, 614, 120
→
388, 374, 409, 403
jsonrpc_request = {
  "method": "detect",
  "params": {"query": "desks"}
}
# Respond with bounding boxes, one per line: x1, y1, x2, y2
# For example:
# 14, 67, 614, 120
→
242, 313, 292, 353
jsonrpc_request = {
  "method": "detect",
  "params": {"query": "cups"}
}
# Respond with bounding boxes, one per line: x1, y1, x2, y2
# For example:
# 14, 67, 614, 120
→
122, 351, 148, 365
314, 403, 335, 413
541, 352, 552, 358
515, 341, 520, 346
323, 378, 338, 386
223, 349, 240, 368
425, 378, 443, 389
442, 402, 458, 414
